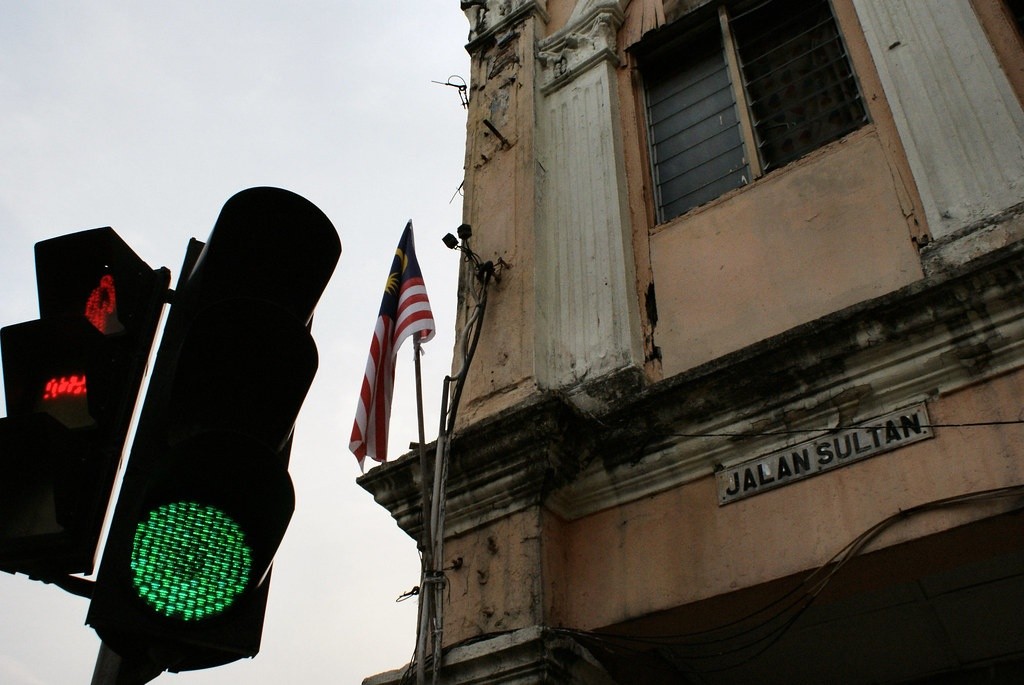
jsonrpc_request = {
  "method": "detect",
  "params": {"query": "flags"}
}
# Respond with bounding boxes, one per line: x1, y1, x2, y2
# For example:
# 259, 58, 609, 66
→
347, 219, 435, 473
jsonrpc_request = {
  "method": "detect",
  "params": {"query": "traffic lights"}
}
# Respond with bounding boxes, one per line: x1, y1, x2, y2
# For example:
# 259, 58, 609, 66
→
1, 186, 341, 685
